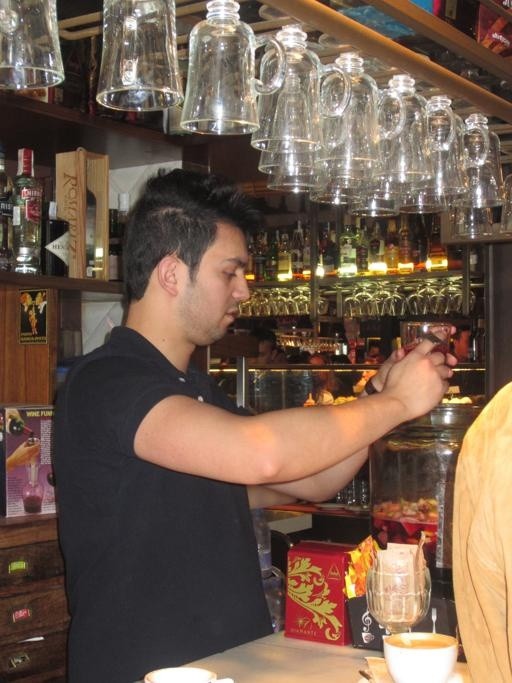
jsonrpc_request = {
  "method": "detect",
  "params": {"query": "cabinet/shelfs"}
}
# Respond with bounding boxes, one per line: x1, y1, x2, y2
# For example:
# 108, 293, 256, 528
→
1, 522, 70, 682
210, 193, 494, 522
0, 89, 211, 292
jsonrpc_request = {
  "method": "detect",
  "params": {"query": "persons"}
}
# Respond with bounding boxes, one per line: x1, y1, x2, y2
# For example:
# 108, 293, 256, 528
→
220, 323, 484, 416
20, 288, 47, 336
5, 408, 41, 472
51, 167, 461, 683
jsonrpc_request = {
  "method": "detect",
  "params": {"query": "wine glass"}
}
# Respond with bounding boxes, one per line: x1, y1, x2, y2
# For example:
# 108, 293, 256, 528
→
345, 281, 477, 316
369, 561, 432, 635
238, 283, 328, 317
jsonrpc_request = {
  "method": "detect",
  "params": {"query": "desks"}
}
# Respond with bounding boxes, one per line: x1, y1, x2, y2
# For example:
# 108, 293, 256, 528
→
133, 630, 471, 683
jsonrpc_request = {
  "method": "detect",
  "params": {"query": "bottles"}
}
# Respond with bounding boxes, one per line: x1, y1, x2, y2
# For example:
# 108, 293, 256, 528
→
1, 149, 16, 272
1, 414, 35, 437
473, 318, 487, 368
277, 333, 352, 368
244, 213, 478, 281
12, 146, 43, 275
22, 438, 44, 514
251, 508, 271, 580
109, 190, 129, 282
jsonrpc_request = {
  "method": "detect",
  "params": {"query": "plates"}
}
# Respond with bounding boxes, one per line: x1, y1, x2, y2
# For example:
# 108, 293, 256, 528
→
358, 675, 465, 683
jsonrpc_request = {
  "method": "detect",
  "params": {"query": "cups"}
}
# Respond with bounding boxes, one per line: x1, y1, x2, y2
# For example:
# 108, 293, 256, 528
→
0, 0, 65, 91
94, 1, 185, 110
382, 633, 461, 683
144, 667, 236, 683
251, 0, 512, 239
180, 1, 287, 136
399, 321, 451, 360
